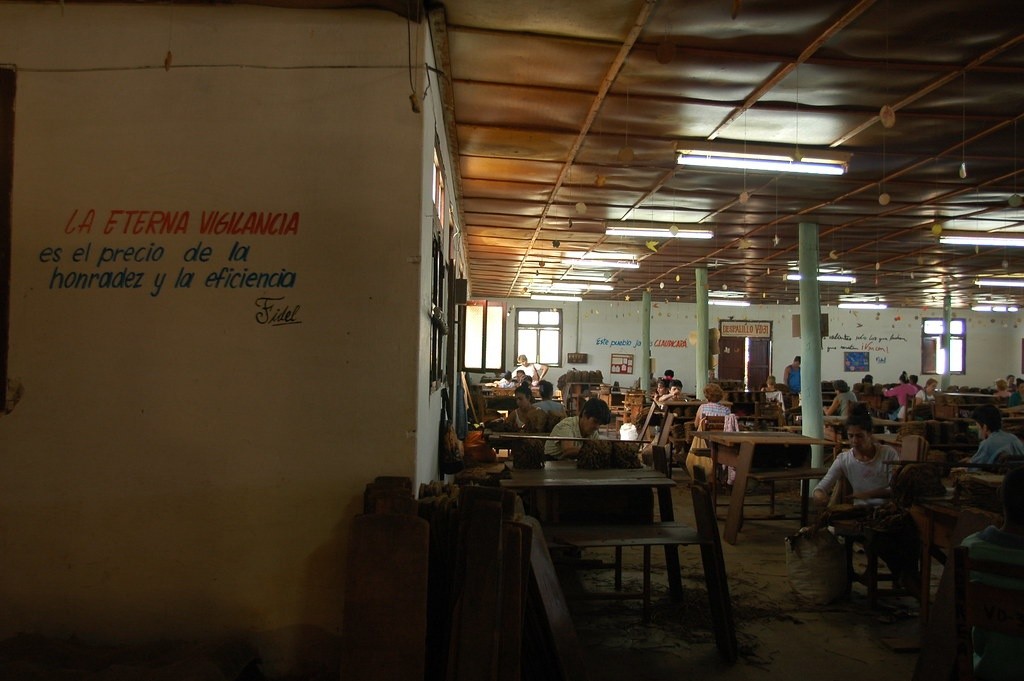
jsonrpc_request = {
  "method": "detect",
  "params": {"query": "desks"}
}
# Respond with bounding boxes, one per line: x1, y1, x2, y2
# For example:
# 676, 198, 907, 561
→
499, 465, 683, 599
690, 429, 838, 528
656, 399, 708, 444
822, 414, 900, 444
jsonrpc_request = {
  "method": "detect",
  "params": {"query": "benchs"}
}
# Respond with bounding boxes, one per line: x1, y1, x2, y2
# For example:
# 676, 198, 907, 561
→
486, 385, 1024, 665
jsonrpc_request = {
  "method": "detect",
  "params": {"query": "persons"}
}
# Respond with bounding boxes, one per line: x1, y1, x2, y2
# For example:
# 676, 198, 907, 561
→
496, 370, 533, 394
694, 383, 731, 428
658, 379, 683, 402
964, 404, 1024, 465
709, 370, 718, 384
784, 356, 801, 406
649, 370, 674, 394
507, 385, 546, 429
760, 376, 779, 392
810, 404, 903, 512
993, 379, 1011, 396
883, 371, 918, 421
959, 467, 1024, 681
542, 397, 611, 461
1006, 375, 1016, 393
914, 378, 938, 406
1007, 378, 1024, 407
822, 379, 858, 416
909, 375, 923, 390
533, 380, 564, 415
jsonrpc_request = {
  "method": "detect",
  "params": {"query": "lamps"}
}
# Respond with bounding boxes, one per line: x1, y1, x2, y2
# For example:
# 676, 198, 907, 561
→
529, 250, 640, 303
673, 140, 852, 176
603, 220, 714, 240
939, 231, 1024, 247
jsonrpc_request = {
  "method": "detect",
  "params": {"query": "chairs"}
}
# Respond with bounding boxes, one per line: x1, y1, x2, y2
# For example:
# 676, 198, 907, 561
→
904, 393, 916, 423
616, 391, 645, 439
753, 400, 783, 431
701, 412, 739, 432
